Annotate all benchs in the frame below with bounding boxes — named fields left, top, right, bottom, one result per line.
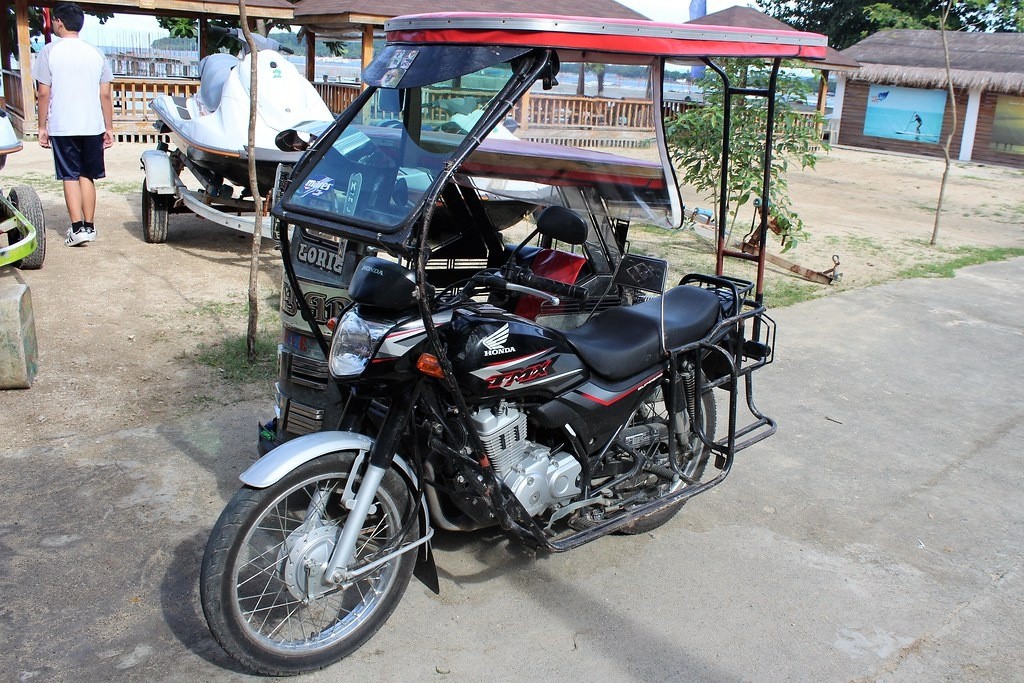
left=482, top=245, right=593, bottom=322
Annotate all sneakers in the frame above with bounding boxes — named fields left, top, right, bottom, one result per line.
left=64, top=226, right=89, bottom=247
left=85, top=226, right=97, bottom=241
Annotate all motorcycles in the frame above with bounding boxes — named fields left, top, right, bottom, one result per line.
left=0, top=61, right=25, bottom=172
left=147, top=22, right=337, bottom=197
left=195, top=8, right=831, bottom=678
left=370, top=97, right=560, bottom=233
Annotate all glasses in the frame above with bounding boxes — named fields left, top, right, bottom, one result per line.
left=52, top=18, right=57, bottom=23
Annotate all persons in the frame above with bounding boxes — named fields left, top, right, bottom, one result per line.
left=31, top=3, right=114, bottom=247
left=685, top=96, right=703, bottom=113
left=909, top=112, right=922, bottom=134
